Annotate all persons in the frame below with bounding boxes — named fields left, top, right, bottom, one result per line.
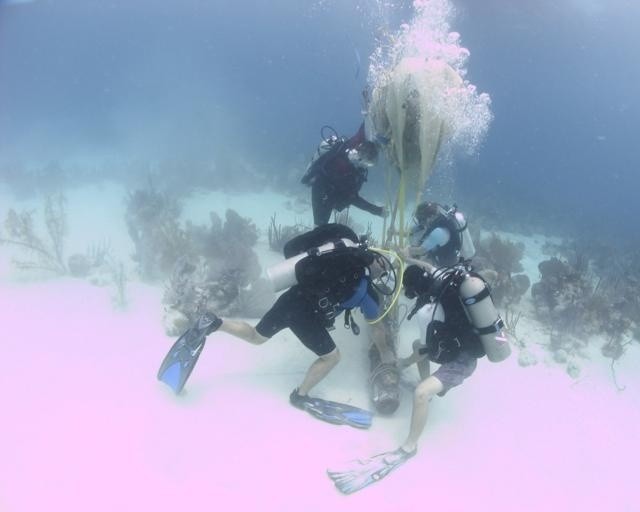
left=382, top=246, right=485, bottom=466
left=307, top=119, right=390, bottom=234
left=384, top=200, right=464, bottom=269
left=185, top=243, right=400, bottom=416
